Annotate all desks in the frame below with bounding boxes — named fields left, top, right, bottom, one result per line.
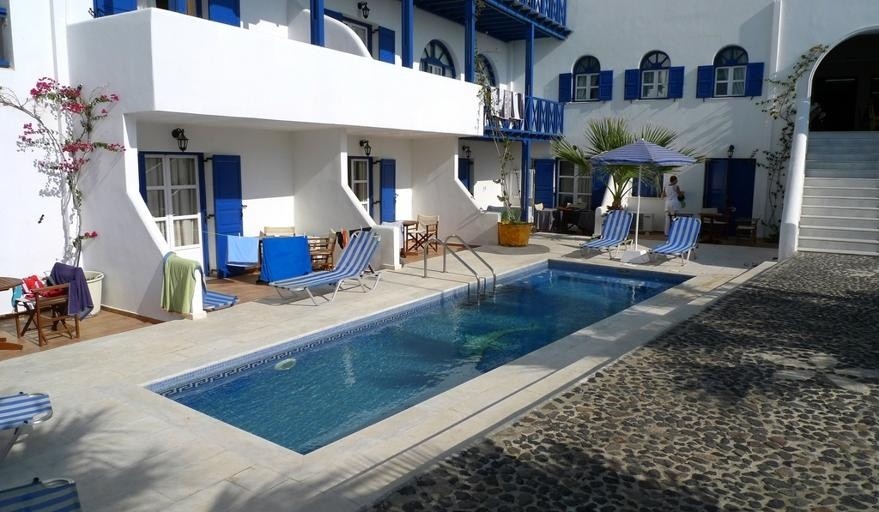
left=0, top=277, right=23, bottom=350
left=392, top=220, right=419, bottom=257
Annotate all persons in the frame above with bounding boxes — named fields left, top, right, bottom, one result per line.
left=659, top=175, right=685, bottom=237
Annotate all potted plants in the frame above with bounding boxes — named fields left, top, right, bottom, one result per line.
left=475, top=44, right=533, bottom=246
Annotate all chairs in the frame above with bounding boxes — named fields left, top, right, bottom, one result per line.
left=579, top=207, right=760, bottom=265
left=0, top=392, right=53, bottom=464
left=408, top=213, right=439, bottom=253
left=259, top=227, right=381, bottom=306
left=204, top=290, right=239, bottom=311
left=0, top=477, right=82, bottom=512
left=13, top=262, right=80, bottom=347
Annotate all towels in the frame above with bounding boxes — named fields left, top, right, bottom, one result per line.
left=48, top=263, right=93, bottom=321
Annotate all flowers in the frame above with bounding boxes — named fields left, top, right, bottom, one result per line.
left=17, top=76, right=126, bottom=267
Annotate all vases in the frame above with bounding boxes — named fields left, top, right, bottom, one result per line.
left=84, top=270, right=104, bottom=319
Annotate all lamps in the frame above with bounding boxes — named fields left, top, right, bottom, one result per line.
left=462, top=145, right=471, bottom=158
left=172, top=128, right=189, bottom=152
left=360, top=140, right=371, bottom=155
left=358, top=2, right=370, bottom=18
left=727, top=145, right=735, bottom=159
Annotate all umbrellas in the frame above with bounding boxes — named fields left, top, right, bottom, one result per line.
left=587, top=136, right=699, bottom=251
